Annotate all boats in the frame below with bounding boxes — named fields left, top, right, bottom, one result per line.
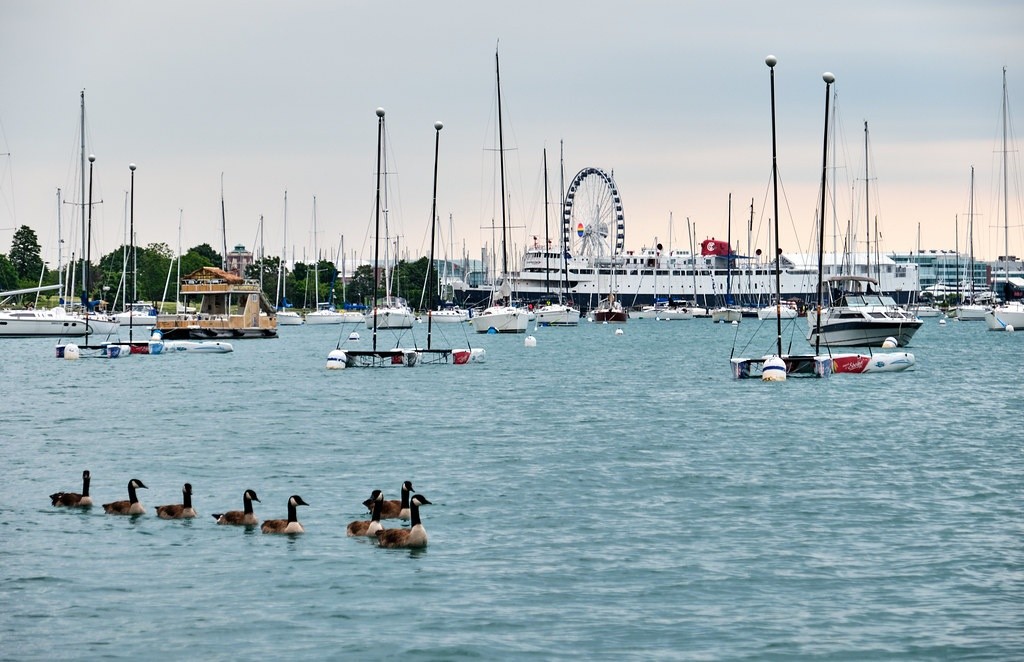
left=0, top=41, right=1023, bottom=382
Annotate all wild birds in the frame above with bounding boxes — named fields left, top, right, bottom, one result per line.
left=211, top=489, right=261, bottom=525
left=374, top=494, right=432, bottom=548
left=154, top=483, right=198, bottom=519
left=362, top=481, right=415, bottom=521
left=346, top=490, right=385, bottom=537
left=49, top=470, right=93, bottom=507
left=261, top=495, right=309, bottom=535
left=101, top=479, right=149, bottom=515
